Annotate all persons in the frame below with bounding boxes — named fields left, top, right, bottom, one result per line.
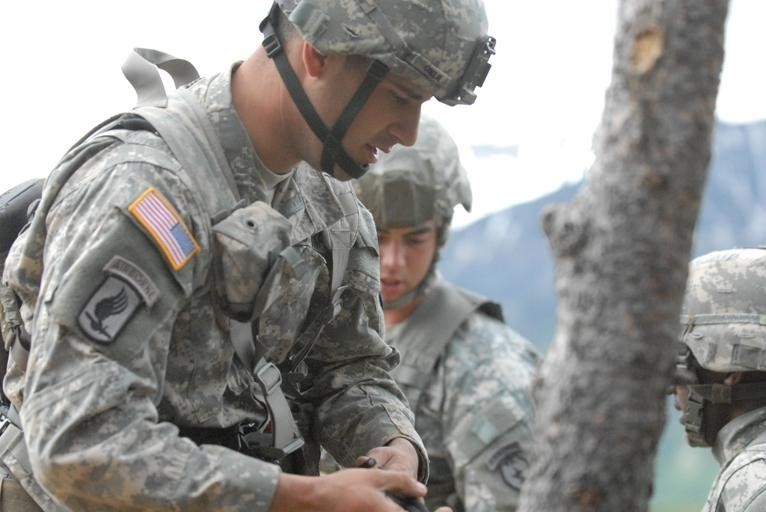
left=666, top=243, right=766, bottom=512
left=318, top=114, right=547, bottom=512
left=0, top=0, right=498, bottom=512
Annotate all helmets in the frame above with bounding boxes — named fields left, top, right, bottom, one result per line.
left=672, top=248, right=766, bottom=373
left=348, top=117, right=471, bottom=226
left=266, top=0, right=497, bottom=106
